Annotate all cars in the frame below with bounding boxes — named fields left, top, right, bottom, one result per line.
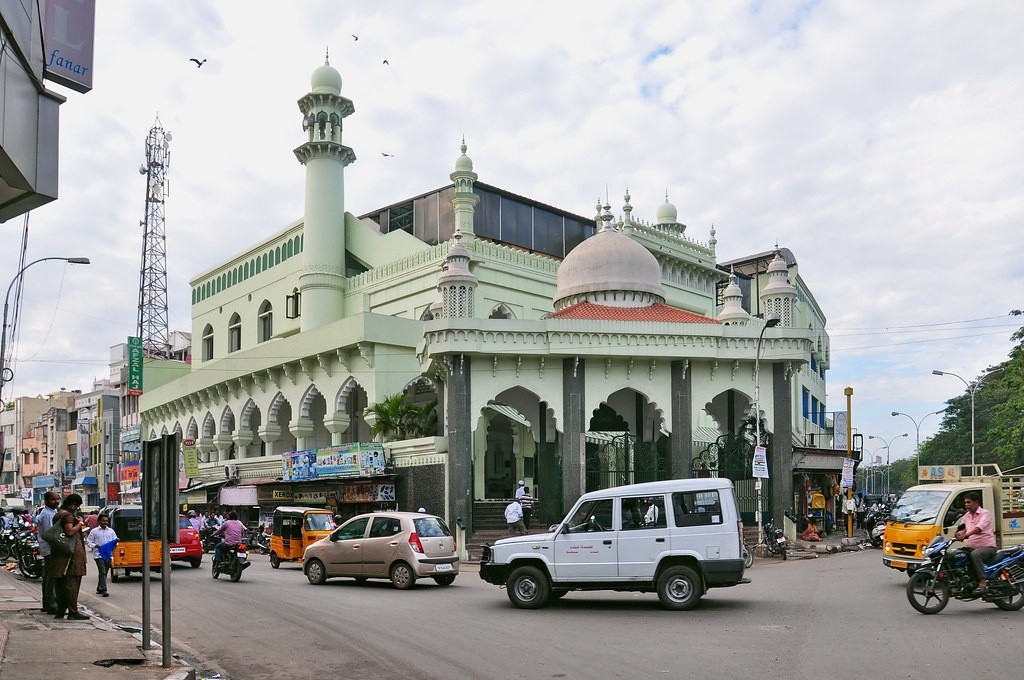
left=302, top=511, right=460, bottom=590
left=170, top=514, right=202, bottom=567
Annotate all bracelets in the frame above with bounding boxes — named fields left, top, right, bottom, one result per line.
left=79, top=522, right=84, bottom=526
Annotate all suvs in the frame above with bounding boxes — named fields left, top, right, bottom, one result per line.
left=478, top=477, right=752, bottom=612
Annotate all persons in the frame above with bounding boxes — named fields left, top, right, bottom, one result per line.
left=519, top=487, right=534, bottom=533
left=188, top=510, right=229, bottom=553
left=53, top=494, right=90, bottom=620
left=76, top=509, right=99, bottom=532
left=86, top=514, right=120, bottom=597
left=505, top=498, right=526, bottom=537
left=213, top=510, right=244, bottom=569
left=842, top=489, right=893, bottom=529
left=515, top=480, right=533, bottom=516
left=955, top=492, right=997, bottom=594
left=644, top=497, right=658, bottom=526
left=694, top=505, right=705, bottom=512
left=35, top=491, right=69, bottom=614
left=0, top=507, right=43, bottom=530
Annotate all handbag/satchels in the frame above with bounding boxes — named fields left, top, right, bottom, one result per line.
left=41, top=513, right=76, bottom=554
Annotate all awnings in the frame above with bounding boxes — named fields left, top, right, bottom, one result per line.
left=486, top=404, right=769, bottom=447
left=116, top=479, right=227, bottom=494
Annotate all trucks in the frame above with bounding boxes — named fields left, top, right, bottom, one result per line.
left=882, top=464, right=1024, bottom=585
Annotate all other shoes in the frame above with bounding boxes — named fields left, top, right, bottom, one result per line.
left=972, top=585, right=986, bottom=594
left=96, top=590, right=102, bottom=594
left=103, top=593, right=109, bottom=596
left=67, top=610, right=90, bottom=620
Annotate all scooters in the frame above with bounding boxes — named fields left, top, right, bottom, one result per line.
left=0, top=505, right=47, bottom=579
left=861, top=493, right=899, bottom=549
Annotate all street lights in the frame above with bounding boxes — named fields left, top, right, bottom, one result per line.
left=868, top=433, right=909, bottom=496
left=0, top=257, right=90, bottom=390
left=863, top=446, right=892, bottom=496
left=755, top=314, right=782, bottom=545
left=932, top=367, right=1004, bottom=474
left=891, top=411, right=942, bottom=484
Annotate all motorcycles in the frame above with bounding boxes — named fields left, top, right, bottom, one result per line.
left=269, top=506, right=336, bottom=569
left=200, top=521, right=273, bottom=582
left=907, top=523, right=1024, bottom=615
left=761, top=515, right=788, bottom=561
left=106, top=505, right=171, bottom=584
